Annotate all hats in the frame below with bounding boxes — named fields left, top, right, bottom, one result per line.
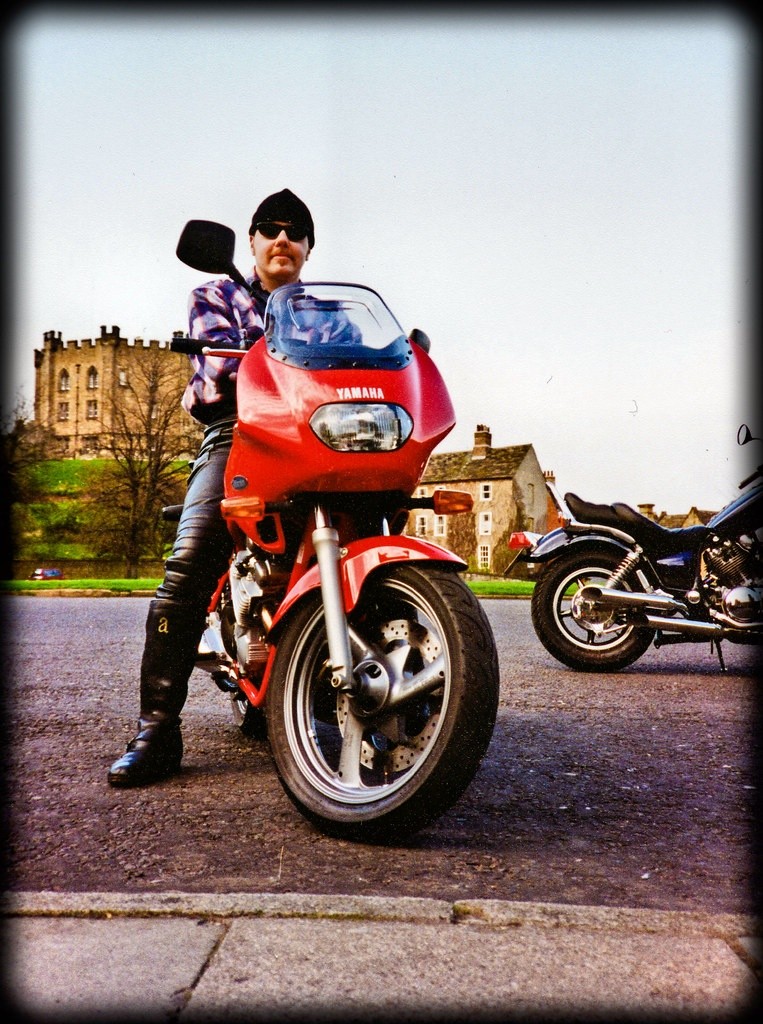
left=252, top=188, right=315, bottom=249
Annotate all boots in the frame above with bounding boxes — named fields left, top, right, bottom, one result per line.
left=107, top=600, right=205, bottom=784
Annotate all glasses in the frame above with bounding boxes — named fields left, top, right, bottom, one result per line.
left=253, top=221, right=311, bottom=249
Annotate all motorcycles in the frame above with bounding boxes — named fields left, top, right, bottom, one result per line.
left=501, top=423, right=762, bottom=674
left=158, top=218, right=503, bottom=847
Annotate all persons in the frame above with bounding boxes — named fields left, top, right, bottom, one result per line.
left=107, top=186, right=363, bottom=791
